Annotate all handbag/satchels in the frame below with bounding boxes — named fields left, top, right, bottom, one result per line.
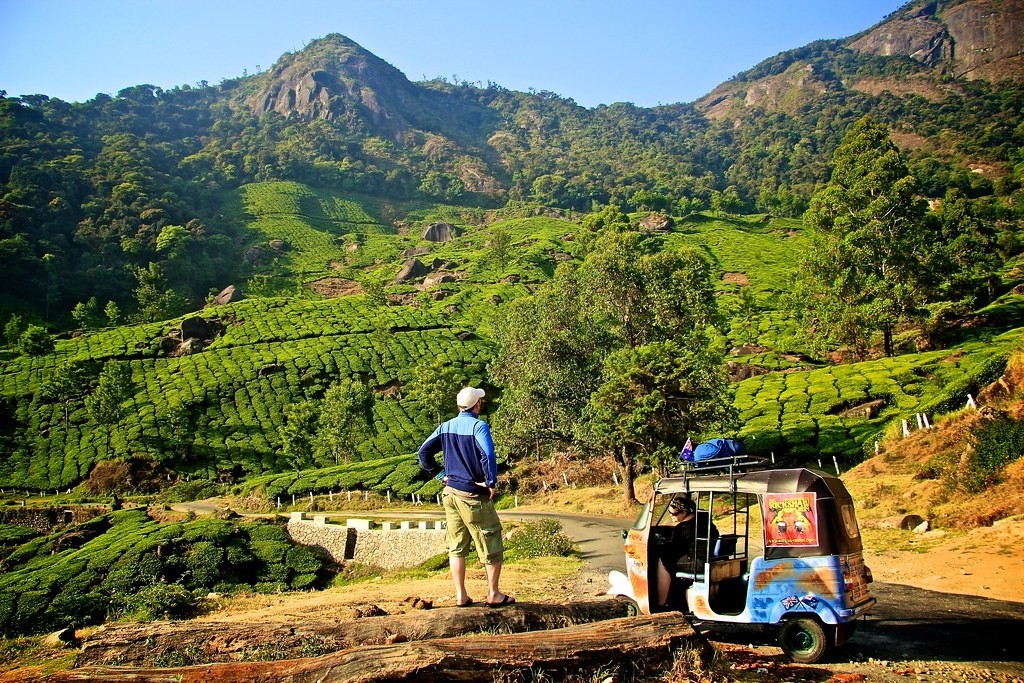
left=677, top=555, right=705, bottom=574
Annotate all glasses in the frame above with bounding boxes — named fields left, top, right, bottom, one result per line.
left=668, top=510, right=683, bottom=516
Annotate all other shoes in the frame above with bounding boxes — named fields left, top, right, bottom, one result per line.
left=650, top=603, right=671, bottom=613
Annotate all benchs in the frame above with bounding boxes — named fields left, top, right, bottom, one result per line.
left=675, top=533, right=737, bottom=580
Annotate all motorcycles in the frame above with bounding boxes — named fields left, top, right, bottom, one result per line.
left=606, top=455, right=879, bottom=666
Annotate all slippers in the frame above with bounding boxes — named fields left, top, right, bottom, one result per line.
left=457, top=596, right=472, bottom=607
left=486, top=595, right=515, bottom=608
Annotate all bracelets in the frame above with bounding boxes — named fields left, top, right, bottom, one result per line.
left=435, top=470, right=445, bottom=483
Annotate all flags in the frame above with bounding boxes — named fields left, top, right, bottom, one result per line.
left=680, top=438, right=694, bottom=463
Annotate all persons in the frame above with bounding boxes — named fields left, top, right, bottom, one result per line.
left=417, top=386, right=515, bottom=607
left=655, top=497, right=720, bottom=611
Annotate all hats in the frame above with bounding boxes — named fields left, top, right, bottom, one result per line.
left=457, top=387, right=485, bottom=412
